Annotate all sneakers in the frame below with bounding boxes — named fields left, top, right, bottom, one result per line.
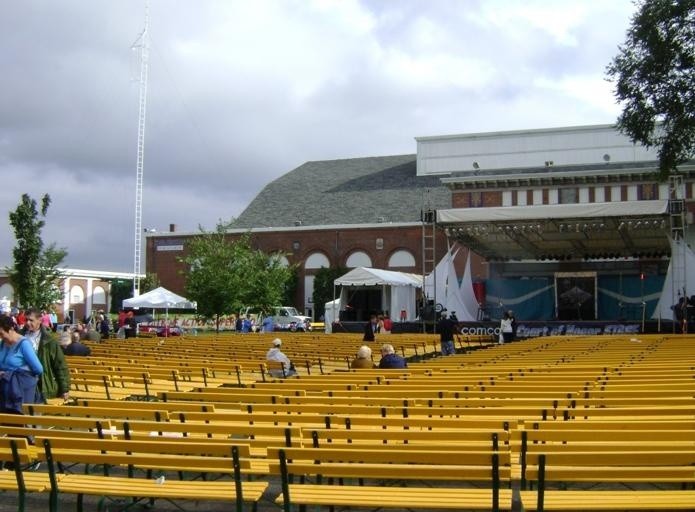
left=33, top=462, right=40, bottom=470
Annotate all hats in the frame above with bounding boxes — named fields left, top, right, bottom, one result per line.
left=272, top=338, right=281, bottom=346
left=358, top=346, right=371, bottom=357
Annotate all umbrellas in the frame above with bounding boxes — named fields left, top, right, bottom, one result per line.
left=122, top=286, right=198, bottom=338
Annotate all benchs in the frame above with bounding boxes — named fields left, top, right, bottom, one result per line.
left=0, top=333, right=695, bottom=507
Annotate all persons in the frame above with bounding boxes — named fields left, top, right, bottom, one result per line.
left=376, top=315, right=385, bottom=334
left=674, top=297, right=687, bottom=334
left=262, top=314, right=274, bottom=333
left=66, top=331, right=92, bottom=357
left=363, top=315, right=377, bottom=342
left=305, top=320, right=312, bottom=332
left=498, top=313, right=513, bottom=344
left=56, top=326, right=72, bottom=348
left=290, top=321, right=296, bottom=333
left=450, top=310, right=458, bottom=330
left=508, top=310, right=517, bottom=343
left=297, top=319, right=307, bottom=332
left=0, top=314, right=44, bottom=472
left=350, top=344, right=378, bottom=369
left=321, top=314, right=326, bottom=322
left=436, top=312, right=458, bottom=355
left=80, top=323, right=91, bottom=340
left=236, top=317, right=244, bottom=333
left=98, top=312, right=110, bottom=338
left=124, top=311, right=137, bottom=339
left=241, top=315, right=251, bottom=333
left=76, top=322, right=84, bottom=335
left=266, top=339, right=298, bottom=378
left=15, top=309, right=70, bottom=464
left=382, top=312, right=393, bottom=333
left=40, top=310, right=54, bottom=329
left=686, top=295, right=695, bottom=334
left=380, top=343, right=407, bottom=369
left=117, top=309, right=127, bottom=336
left=424, top=299, right=436, bottom=334
left=49, top=310, right=59, bottom=332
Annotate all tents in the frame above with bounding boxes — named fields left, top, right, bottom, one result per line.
left=333, top=266, right=433, bottom=322
left=325, top=298, right=340, bottom=333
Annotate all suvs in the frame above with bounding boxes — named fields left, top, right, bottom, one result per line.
left=244, top=306, right=314, bottom=333
